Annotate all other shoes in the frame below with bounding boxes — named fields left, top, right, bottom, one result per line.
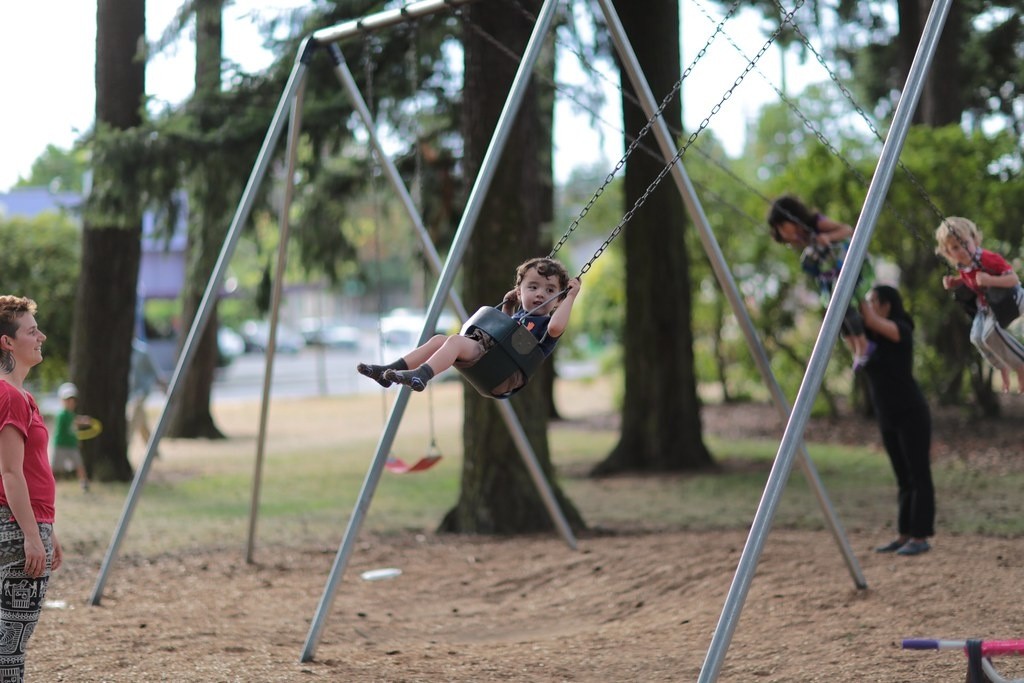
left=877, top=539, right=909, bottom=551
left=897, top=541, right=929, bottom=554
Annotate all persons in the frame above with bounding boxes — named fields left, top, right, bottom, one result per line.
left=48, top=382, right=98, bottom=501
left=934, top=216, right=1024, bottom=394
left=125, top=339, right=169, bottom=458
left=357, top=257, right=582, bottom=400
left=0, top=292, right=64, bottom=683
left=767, top=197, right=937, bottom=556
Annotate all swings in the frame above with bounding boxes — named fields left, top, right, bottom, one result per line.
left=696, top=0, right=1019, bottom=333
left=443, top=1, right=874, bottom=301
left=457, top=0, right=811, bottom=398
left=361, top=22, right=443, bottom=475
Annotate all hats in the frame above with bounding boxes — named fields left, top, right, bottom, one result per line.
left=58, top=381, right=78, bottom=400
